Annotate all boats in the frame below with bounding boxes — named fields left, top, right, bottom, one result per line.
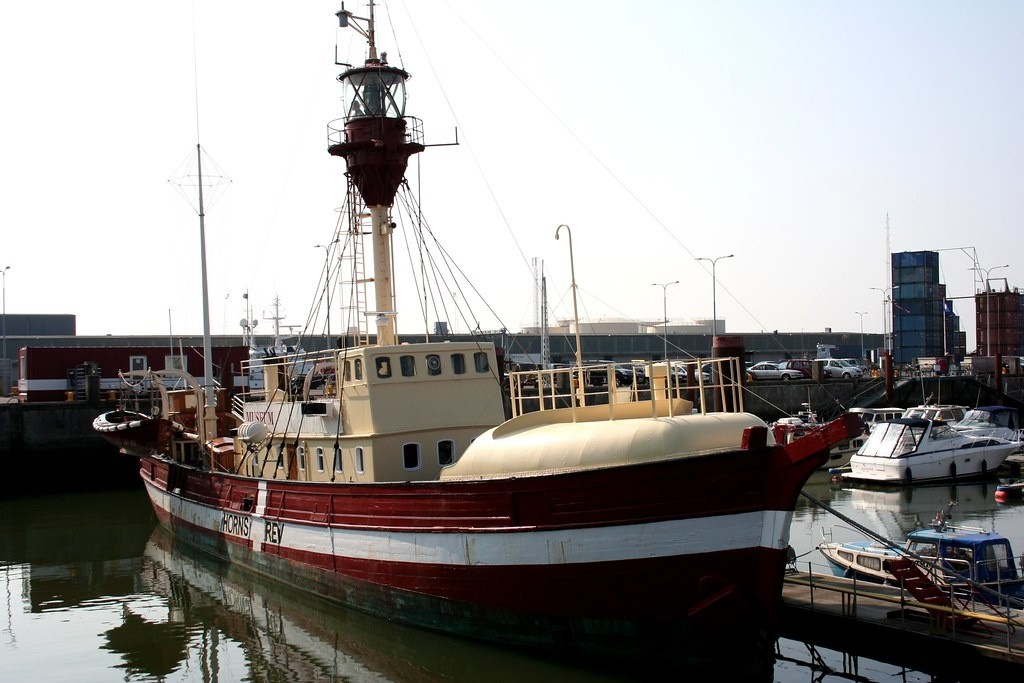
left=787, top=393, right=1024, bottom=454
left=92, top=410, right=184, bottom=458
left=829, top=418, right=1024, bottom=486
left=818, top=502, right=1024, bottom=611
left=139, top=523, right=608, bottom=683
left=118, top=0, right=865, bottom=670
left=842, top=478, right=1010, bottom=543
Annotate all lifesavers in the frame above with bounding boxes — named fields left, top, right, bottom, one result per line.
left=129, top=381, right=144, bottom=396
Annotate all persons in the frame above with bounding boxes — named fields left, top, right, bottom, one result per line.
left=515, top=366, right=524, bottom=396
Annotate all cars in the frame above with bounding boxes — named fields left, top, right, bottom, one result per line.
left=814, top=359, right=863, bottom=379
left=700, top=363, right=757, bottom=386
left=572, top=360, right=639, bottom=386
left=670, top=366, right=710, bottom=383
left=785, top=359, right=813, bottom=378
left=746, top=363, right=803, bottom=380
left=842, top=359, right=868, bottom=372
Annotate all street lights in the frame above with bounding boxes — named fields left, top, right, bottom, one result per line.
left=855, top=312, right=868, bottom=357
left=870, top=286, right=899, bottom=351
left=554, top=224, right=585, bottom=406
left=652, top=281, right=679, bottom=360
left=314, top=239, right=340, bottom=350
left=696, top=255, right=734, bottom=336
left=0, top=266, right=10, bottom=397
left=968, top=265, right=1009, bottom=357
